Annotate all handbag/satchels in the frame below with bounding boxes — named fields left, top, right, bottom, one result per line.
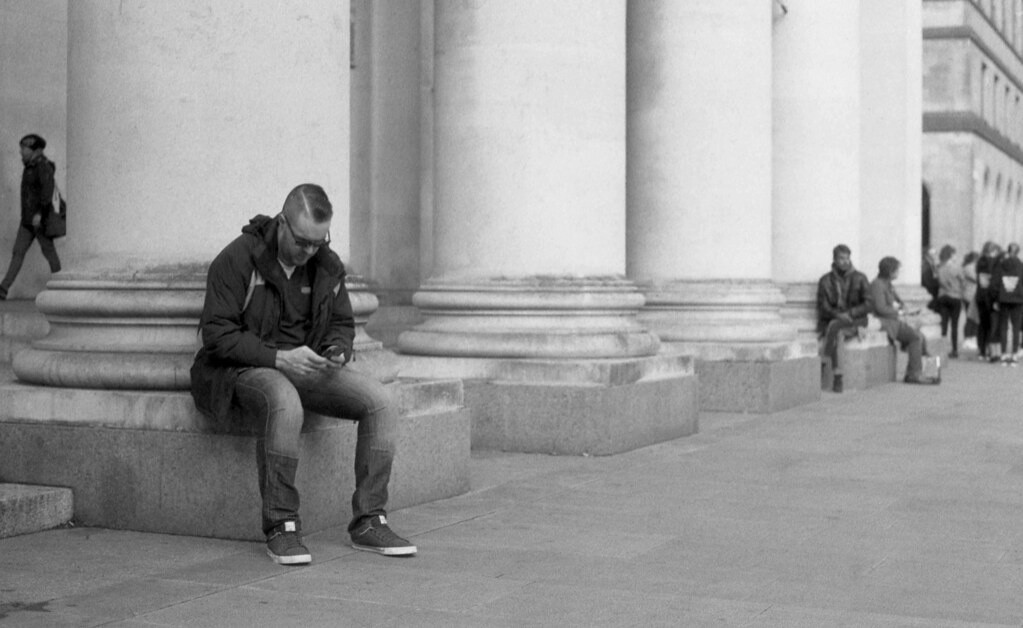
left=42, top=193, right=67, bottom=237
left=920, top=354, right=941, bottom=383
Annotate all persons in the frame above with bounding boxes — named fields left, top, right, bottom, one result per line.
left=922, top=241, right=1023, bottom=366
left=816, top=244, right=873, bottom=392
left=870, top=256, right=941, bottom=384
left=190, top=184, right=417, bottom=565
left=0, top=134, right=61, bottom=300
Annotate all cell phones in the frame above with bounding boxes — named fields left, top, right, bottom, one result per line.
left=326, top=345, right=348, bottom=360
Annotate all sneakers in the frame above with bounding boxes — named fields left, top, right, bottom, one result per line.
left=264, top=520, right=311, bottom=564
left=349, top=514, right=416, bottom=555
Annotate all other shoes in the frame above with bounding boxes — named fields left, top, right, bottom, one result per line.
left=905, top=374, right=933, bottom=384
left=948, top=350, right=959, bottom=358
left=0, top=286, right=8, bottom=299
left=941, top=324, right=947, bottom=336
left=832, top=374, right=842, bottom=392
left=976, top=349, right=1023, bottom=363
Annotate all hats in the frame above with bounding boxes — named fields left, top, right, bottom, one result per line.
left=20, top=133, right=47, bottom=151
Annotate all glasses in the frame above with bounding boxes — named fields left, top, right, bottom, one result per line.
left=284, top=215, right=331, bottom=251
